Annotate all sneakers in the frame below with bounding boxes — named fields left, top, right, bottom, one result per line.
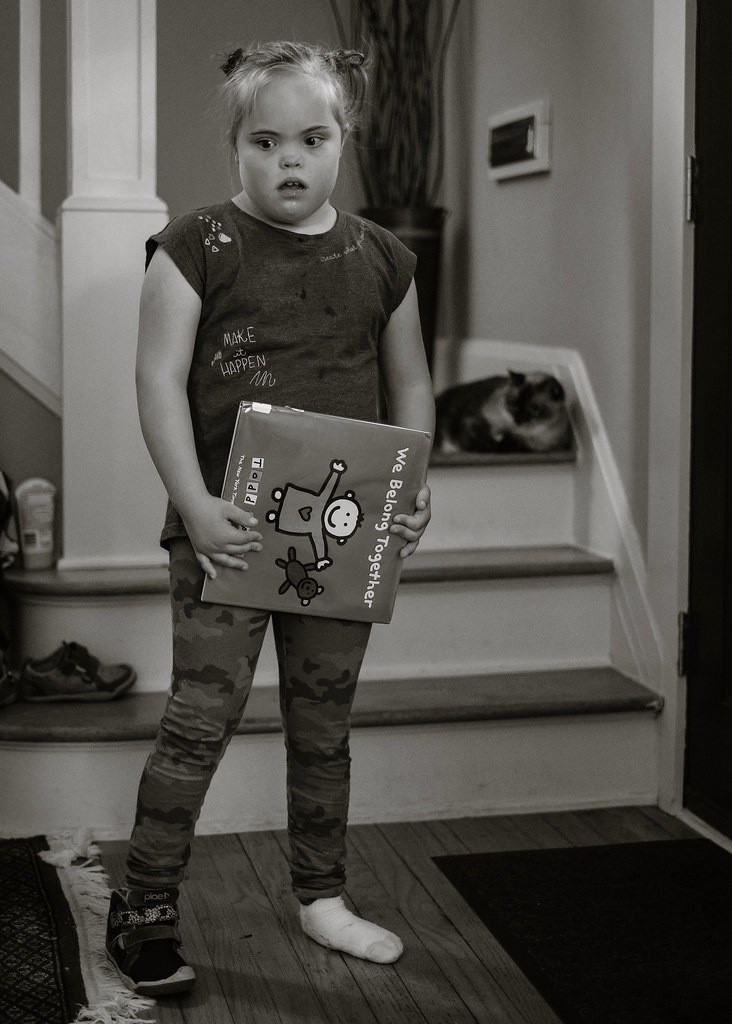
left=20, top=640, right=136, bottom=703
left=0, top=666, right=16, bottom=706
left=106, top=887, right=196, bottom=996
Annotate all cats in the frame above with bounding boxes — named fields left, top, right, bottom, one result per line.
left=431, top=367, right=570, bottom=452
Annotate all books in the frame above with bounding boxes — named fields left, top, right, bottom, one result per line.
left=201, top=400, right=433, bottom=625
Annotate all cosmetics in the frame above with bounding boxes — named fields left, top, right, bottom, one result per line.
left=15, top=477, right=54, bottom=572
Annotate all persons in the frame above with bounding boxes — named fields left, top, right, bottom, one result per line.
left=106, top=38, right=436, bottom=992
